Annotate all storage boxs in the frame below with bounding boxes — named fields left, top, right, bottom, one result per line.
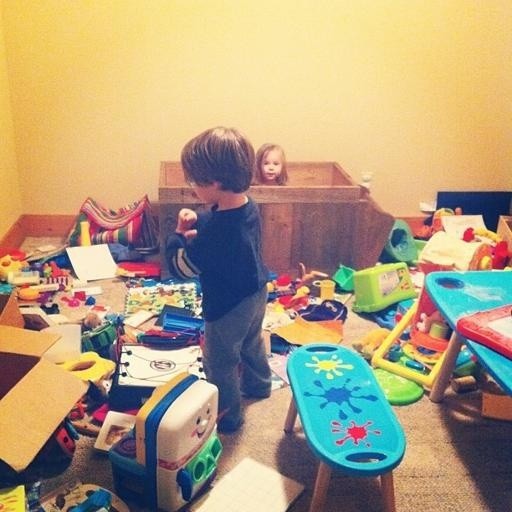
left=156, top=152, right=399, bottom=281
left=0, top=322, right=89, bottom=475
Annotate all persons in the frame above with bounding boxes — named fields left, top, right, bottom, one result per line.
left=165, top=126, right=272, bottom=434
left=252, top=143, right=289, bottom=187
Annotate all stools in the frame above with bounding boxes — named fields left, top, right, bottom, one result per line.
left=281, top=341, right=406, bottom=511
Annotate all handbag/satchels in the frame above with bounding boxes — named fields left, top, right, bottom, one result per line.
left=68, top=197, right=160, bottom=256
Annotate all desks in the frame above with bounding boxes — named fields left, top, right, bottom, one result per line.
left=423, top=269, right=512, bottom=404
